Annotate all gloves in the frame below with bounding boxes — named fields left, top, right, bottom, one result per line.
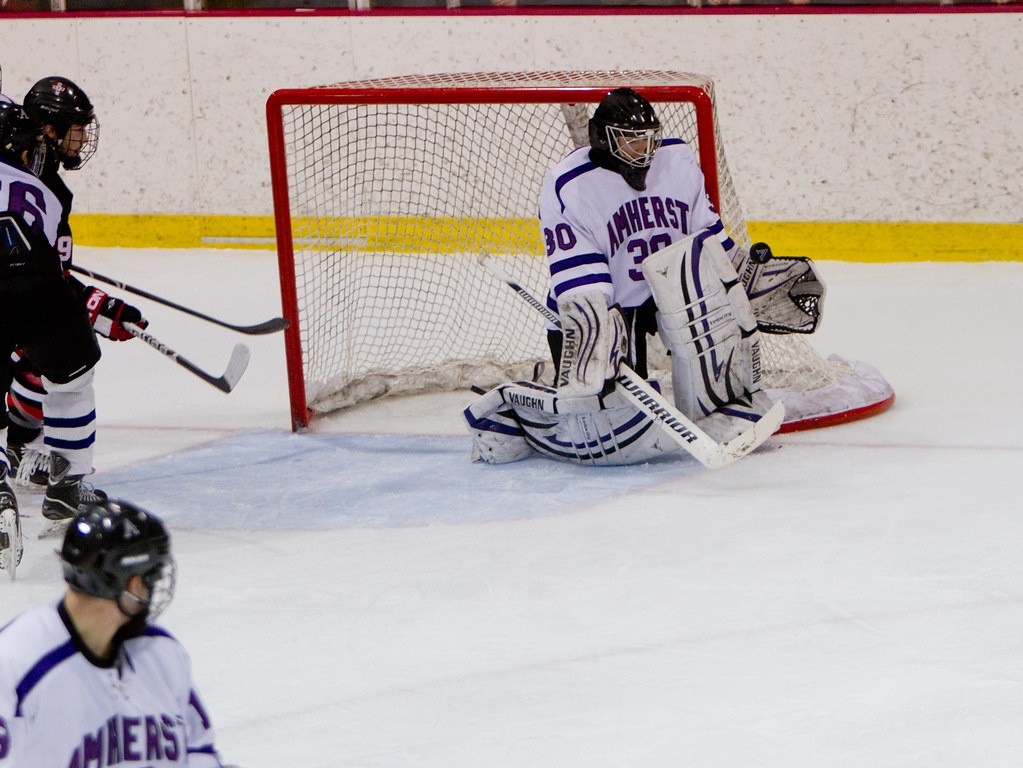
left=83, top=286, right=149, bottom=342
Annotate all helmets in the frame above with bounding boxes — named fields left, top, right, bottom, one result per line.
left=60, top=499, right=176, bottom=623
left=588, top=88, right=663, bottom=192
left=0, top=101, right=47, bottom=177
left=24, top=77, right=100, bottom=169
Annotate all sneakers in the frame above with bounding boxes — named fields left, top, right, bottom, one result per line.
left=0, top=461, right=24, bottom=579
left=38, top=449, right=108, bottom=541
left=5, top=411, right=51, bottom=492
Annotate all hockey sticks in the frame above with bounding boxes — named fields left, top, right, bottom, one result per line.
left=476, top=250, right=787, bottom=471
left=68, top=263, right=292, bottom=337
left=121, top=319, right=251, bottom=394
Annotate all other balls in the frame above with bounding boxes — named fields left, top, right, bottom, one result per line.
left=749, top=242, right=772, bottom=264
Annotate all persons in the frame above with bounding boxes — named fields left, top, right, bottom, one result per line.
left=0, top=498, right=231, bottom=768
left=463, top=86, right=823, bottom=466
left=0, top=77, right=147, bottom=582
